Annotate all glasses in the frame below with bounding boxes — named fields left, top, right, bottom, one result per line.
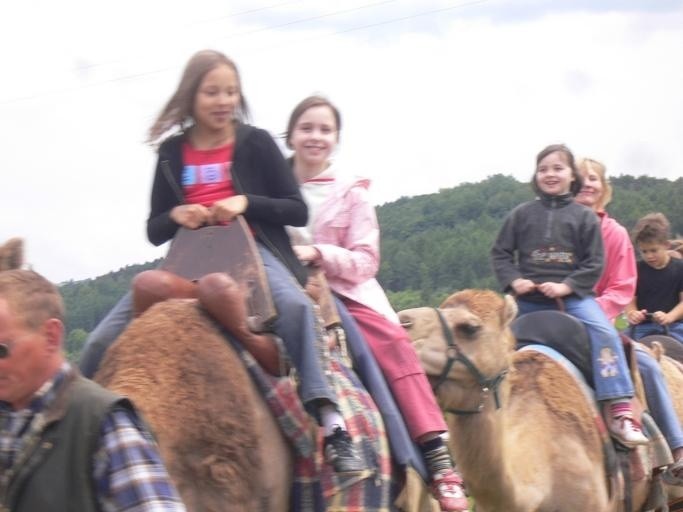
left=0, top=333, right=36, bottom=360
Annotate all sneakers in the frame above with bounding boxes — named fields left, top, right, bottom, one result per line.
left=609, top=414, right=651, bottom=446
left=322, top=425, right=364, bottom=473
left=431, top=468, right=468, bottom=512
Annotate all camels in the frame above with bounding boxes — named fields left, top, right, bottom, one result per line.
left=393, top=287, right=683, bottom=510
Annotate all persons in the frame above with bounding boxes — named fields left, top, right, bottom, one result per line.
left=620, top=212, right=683, bottom=345
left=75, top=48, right=367, bottom=476
left=275, top=94, right=469, bottom=511
left=572, top=156, right=683, bottom=475
left=0, top=268, right=188, bottom=511
left=490, top=142, right=651, bottom=448
